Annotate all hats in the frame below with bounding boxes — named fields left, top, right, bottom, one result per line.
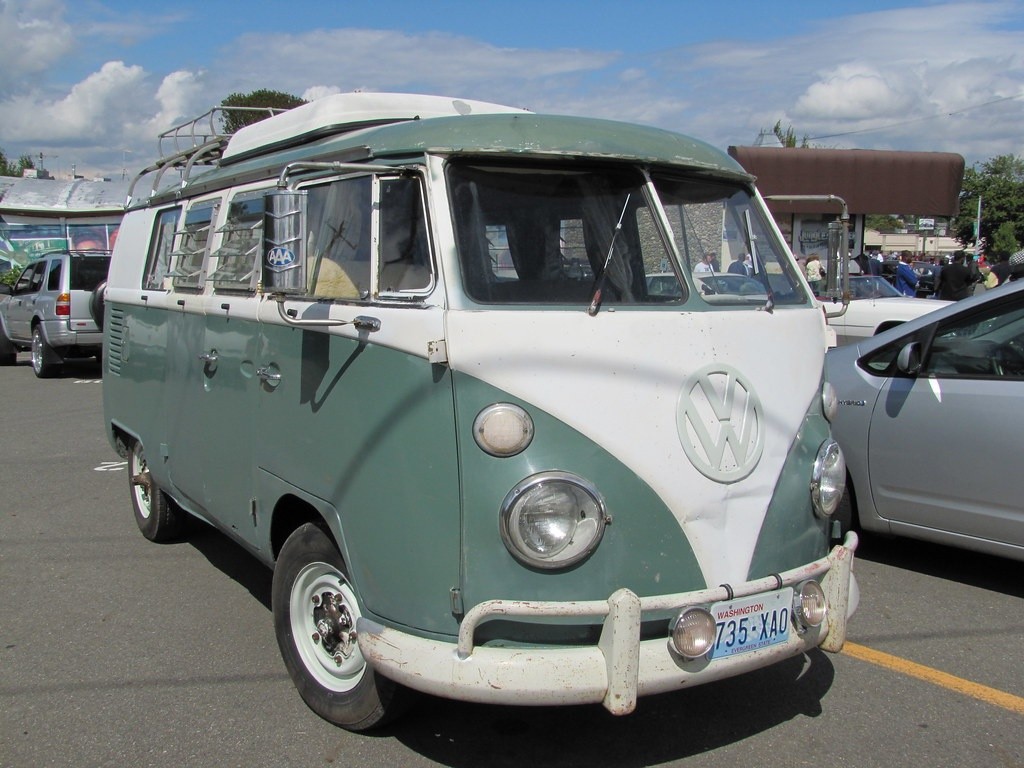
left=1009, top=251, right=1024, bottom=266
left=953, top=250, right=966, bottom=258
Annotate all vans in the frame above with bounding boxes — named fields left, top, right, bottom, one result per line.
left=95, top=90, right=866, bottom=734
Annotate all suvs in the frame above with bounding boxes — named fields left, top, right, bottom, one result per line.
left=0, top=246, right=114, bottom=379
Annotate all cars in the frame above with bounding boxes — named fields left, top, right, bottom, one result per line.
left=790, top=273, right=959, bottom=339
left=825, top=274, right=1023, bottom=562
left=644, top=271, right=770, bottom=304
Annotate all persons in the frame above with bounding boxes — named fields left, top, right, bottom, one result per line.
left=868, top=246, right=1024, bottom=301
left=791, top=253, right=825, bottom=297
left=72, top=230, right=107, bottom=254
left=694, top=252, right=752, bottom=277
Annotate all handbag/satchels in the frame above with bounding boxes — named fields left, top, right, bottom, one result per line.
left=819, top=261, right=826, bottom=277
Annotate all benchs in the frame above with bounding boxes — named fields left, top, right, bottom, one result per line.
left=306, top=257, right=430, bottom=300
